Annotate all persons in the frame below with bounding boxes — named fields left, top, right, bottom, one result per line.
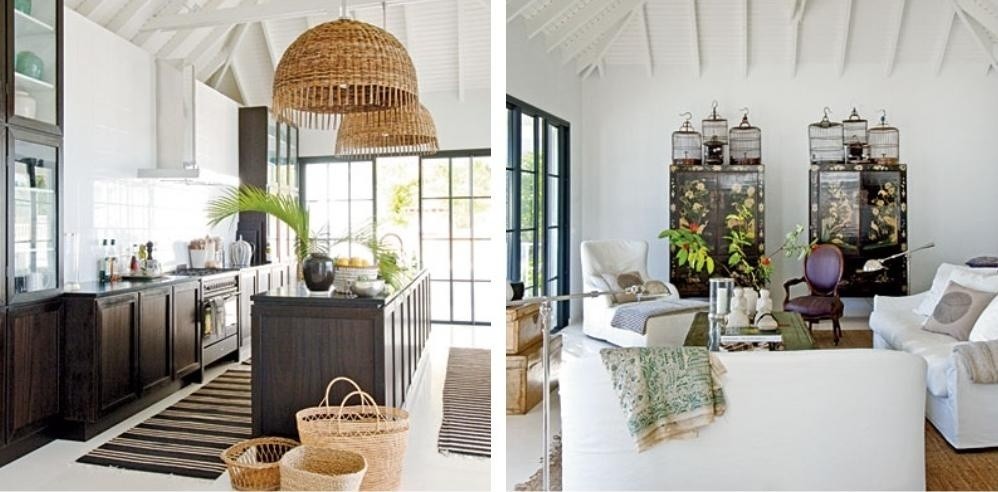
left=728, top=287, right=749, bottom=324
left=756, top=289, right=772, bottom=323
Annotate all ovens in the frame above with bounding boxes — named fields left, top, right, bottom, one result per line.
left=202, top=288, right=238, bottom=348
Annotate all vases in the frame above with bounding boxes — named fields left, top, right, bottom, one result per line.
left=737, top=286, right=758, bottom=321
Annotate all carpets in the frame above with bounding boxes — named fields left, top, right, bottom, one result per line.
left=434, top=344, right=491, bottom=461
left=74, top=369, right=253, bottom=480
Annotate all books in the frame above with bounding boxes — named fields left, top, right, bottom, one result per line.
left=721, top=325, right=783, bottom=342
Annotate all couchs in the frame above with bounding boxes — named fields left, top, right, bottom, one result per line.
left=559, top=350, right=928, bottom=490
left=867, top=254, right=998, bottom=453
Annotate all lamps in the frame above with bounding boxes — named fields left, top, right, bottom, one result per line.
left=333, top=1, right=439, bottom=160
left=273, top=1, right=418, bottom=130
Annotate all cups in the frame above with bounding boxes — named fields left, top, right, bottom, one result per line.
left=14, top=273, right=29, bottom=294
left=27, top=272, right=50, bottom=292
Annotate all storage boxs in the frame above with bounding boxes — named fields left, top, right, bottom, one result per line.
left=506, top=299, right=563, bottom=415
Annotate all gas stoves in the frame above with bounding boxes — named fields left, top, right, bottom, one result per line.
left=164, top=267, right=239, bottom=295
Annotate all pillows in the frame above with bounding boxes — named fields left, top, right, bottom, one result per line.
left=592, top=267, right=680, bottom=304
left=922, top=279, right=998, bottom=341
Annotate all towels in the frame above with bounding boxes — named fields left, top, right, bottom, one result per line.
left=599, top=346, right=727, bottom=452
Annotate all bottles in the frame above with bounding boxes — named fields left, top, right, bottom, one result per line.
left=98, top=238, right=162, bottom=287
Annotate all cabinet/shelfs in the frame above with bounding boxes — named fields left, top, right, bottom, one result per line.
left=668, top=164, right=908, bottom=299
left=167, top=281, right=201, bottom=392
left=58, top=294, right=138, bottom=442
left=0, top=0, right=64, bottom=468
left=238, top=106, right=299, bottom=363
left=133, top=286, right=173, bottom=414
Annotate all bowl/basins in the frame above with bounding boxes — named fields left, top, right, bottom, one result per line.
left=333, top=266, right=379, bottom=292
left=346, top=279, right=385, bottom=298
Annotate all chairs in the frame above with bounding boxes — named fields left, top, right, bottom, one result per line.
left=581, top=237, right=719, bottom=349
left=783, top=241, right=845, bottom=346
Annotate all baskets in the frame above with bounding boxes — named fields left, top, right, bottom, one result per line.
left=219, top=437, right=300, bottom=491
left=279, top=446, right=367, bottom=490
left=294, top=377, right=410, bottom=490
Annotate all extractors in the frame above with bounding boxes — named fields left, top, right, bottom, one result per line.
left=138, top=62, right=241, bottom=187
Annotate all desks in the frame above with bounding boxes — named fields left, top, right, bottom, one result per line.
left=682, top=312, right=817, bottom=350
left=250, top=267, right=430, bottom=439
